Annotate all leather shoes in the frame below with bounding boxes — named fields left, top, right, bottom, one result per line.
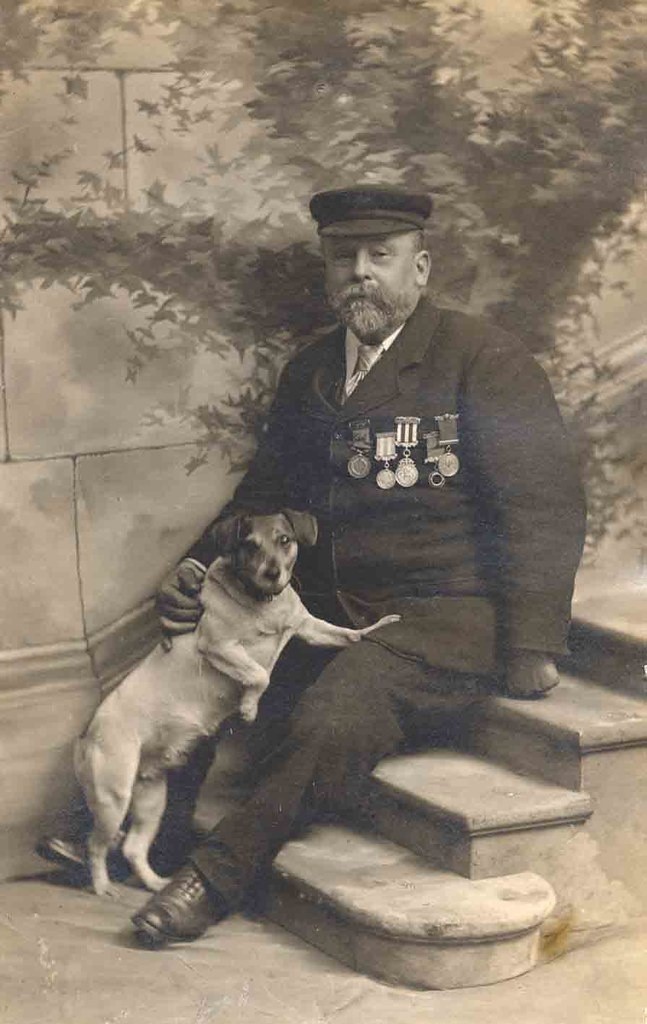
left=131, top=857, right=225, bottom=944
left=36, top=832, right=184, bottom=872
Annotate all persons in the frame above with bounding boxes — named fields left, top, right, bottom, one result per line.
left=37, top=185, right=588, bottom=943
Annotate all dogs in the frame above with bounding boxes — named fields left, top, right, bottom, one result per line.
left=71, top=506, right=403, bottom=901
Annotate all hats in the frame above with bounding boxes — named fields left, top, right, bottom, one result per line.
left=309, top=187, right=432, bottom=236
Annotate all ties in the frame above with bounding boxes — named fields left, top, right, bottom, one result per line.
left=342, top=345, right=383, bottom=407
